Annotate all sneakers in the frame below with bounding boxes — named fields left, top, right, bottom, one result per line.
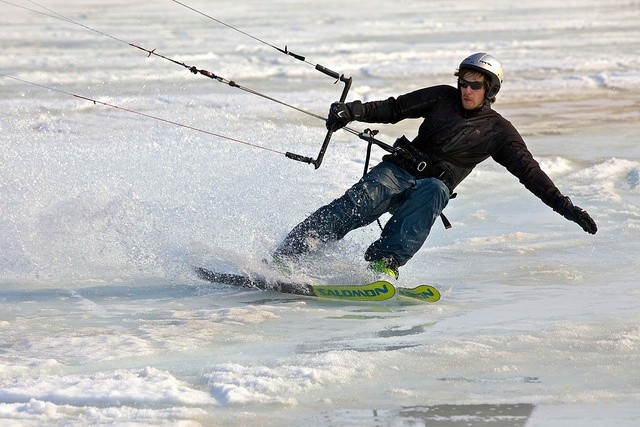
left=367, top=258, right=400, bottom=281
left=263, top=255, right=295, bottom=280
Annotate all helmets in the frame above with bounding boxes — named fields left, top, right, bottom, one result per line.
left=454, top=53, right=504, bottom=96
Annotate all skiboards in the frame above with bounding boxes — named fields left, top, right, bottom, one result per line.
left=187, top=265, right=442, bottom=304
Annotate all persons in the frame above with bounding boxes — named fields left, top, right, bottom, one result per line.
left=260, top=50, right=597, bottom=281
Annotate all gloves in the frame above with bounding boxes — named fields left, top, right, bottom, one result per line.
left=326, top=100, right=364, bottom=133
left=553, top=195, right=598, bottom=235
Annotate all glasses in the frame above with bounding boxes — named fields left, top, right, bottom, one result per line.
left=458, top=78, right=484, bottom=89
